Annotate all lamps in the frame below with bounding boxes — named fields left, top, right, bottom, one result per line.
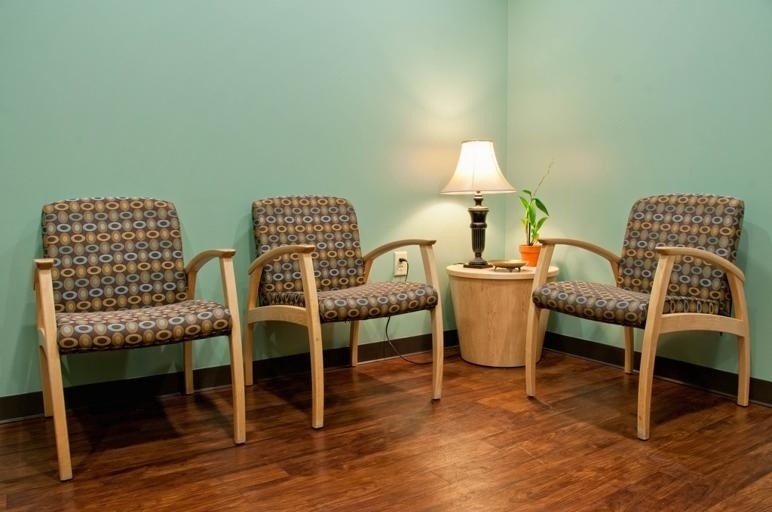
left=437, top=137, right=517, bottom=270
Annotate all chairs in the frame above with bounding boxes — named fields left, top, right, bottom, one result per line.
left=238, top=190, right=446, bottom=429
left=520, top=189, right=755, bottom=442
left=27, top=190, right=249, bottom=482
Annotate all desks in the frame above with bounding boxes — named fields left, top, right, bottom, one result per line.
left=446, top=260, right=564, bottom=371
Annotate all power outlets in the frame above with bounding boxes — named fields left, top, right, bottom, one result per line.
left=393, top=248, right=410, bottom=278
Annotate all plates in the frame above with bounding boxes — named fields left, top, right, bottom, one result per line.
left=486, top=259, right=529, bottom=267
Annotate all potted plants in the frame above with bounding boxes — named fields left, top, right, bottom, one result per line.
left=512, top=185, right=552, bottom=267
